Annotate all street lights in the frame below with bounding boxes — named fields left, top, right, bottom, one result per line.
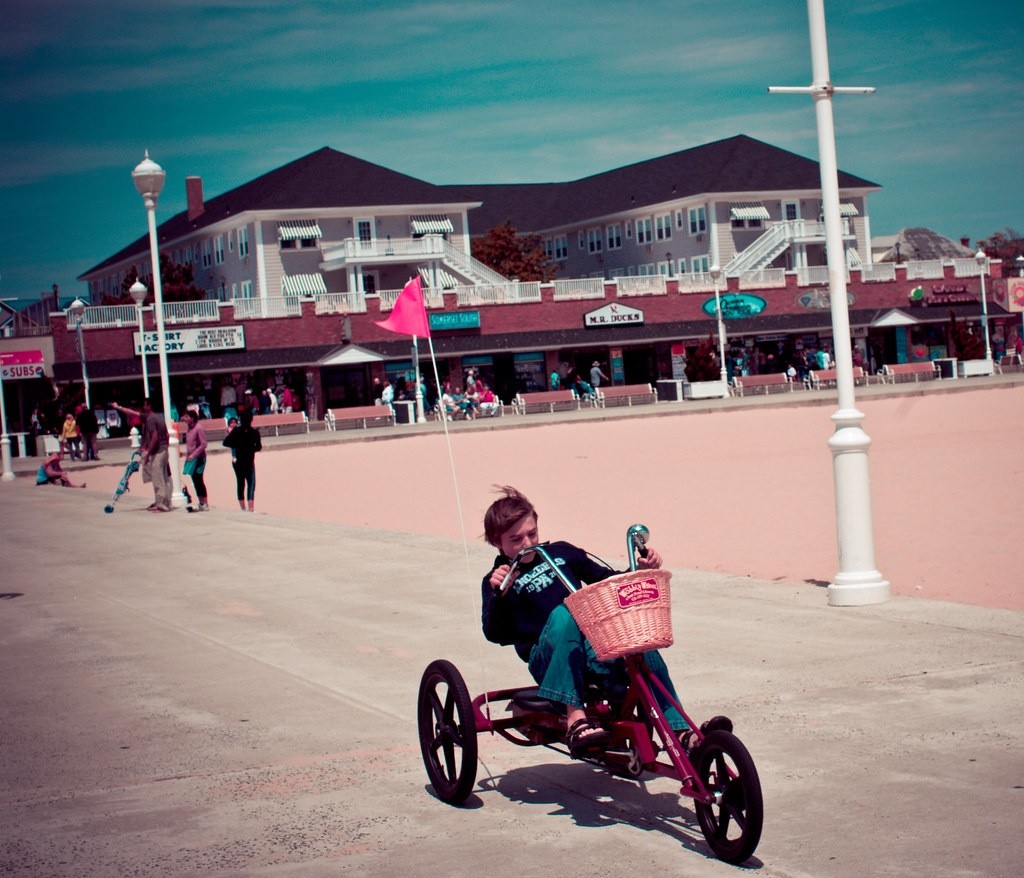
left=129, top=278, right=150, bottom=402
left=710, top=265, right=732, bottom=398
left=973, top=250, right=996, bottom=375
left=69, top=296, right=90, bottom=409
left=131, top=157, right=184, bottom=507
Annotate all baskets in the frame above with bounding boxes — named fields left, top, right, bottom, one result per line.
left=564, top=569, right=674, bottom=660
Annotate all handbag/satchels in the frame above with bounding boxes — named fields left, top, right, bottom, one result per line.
left=142, top=455, right=155, bottom=484
left=182, top=459, right=197, bottom=476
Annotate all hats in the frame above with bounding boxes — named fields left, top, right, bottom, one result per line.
left=592, top=361, right=600, bottom=367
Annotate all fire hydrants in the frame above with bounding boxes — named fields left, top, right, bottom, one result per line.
left=126, top=425, right=142, bottom=448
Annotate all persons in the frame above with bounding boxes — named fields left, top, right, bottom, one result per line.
left=374, top=369, right=499, bottom=422
left=707, top=341, right=864, bottom=388
left=225, top=417, right=239, bottom=461
left=177, top=374, right=295, bottom=421
left=29, top=397, right=173, bottom=510
left=481, top=483, right=732, bottom=755
left=223, top=409, right=263, bottom=512
left=550, top=358, right=609, bottom=403
left=184, top=410, right=209, bottom=510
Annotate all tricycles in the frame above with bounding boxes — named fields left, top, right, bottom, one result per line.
left=416, top=522, right=765, bottom=864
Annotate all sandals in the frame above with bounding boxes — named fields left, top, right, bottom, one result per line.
left=680, top=716, right=733, bottom=758
left=564, top=717, right=608, bottom=760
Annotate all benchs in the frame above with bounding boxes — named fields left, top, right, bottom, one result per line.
left=729, top=372, right=794, bottom=398
left=803, top=367, right=869, bottom=391
left=251, top=411, right=309, bottom=437
left=324, top=402, right=396, bottom=432
left=877, top=361, right=942, bottom=386
left=511, top=389, right=581, bottom=416
left=175, top=417, right=228, bottom=434
left=590, top=382, right=658, bottom=408
left=434, top=394, right=505, bottom=421
left=993, top=354, right=1024, bottom=375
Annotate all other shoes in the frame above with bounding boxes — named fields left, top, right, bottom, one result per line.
left=195, top=503, right=209, bottom=513
left=146, top=504, right=165, bottom=511
